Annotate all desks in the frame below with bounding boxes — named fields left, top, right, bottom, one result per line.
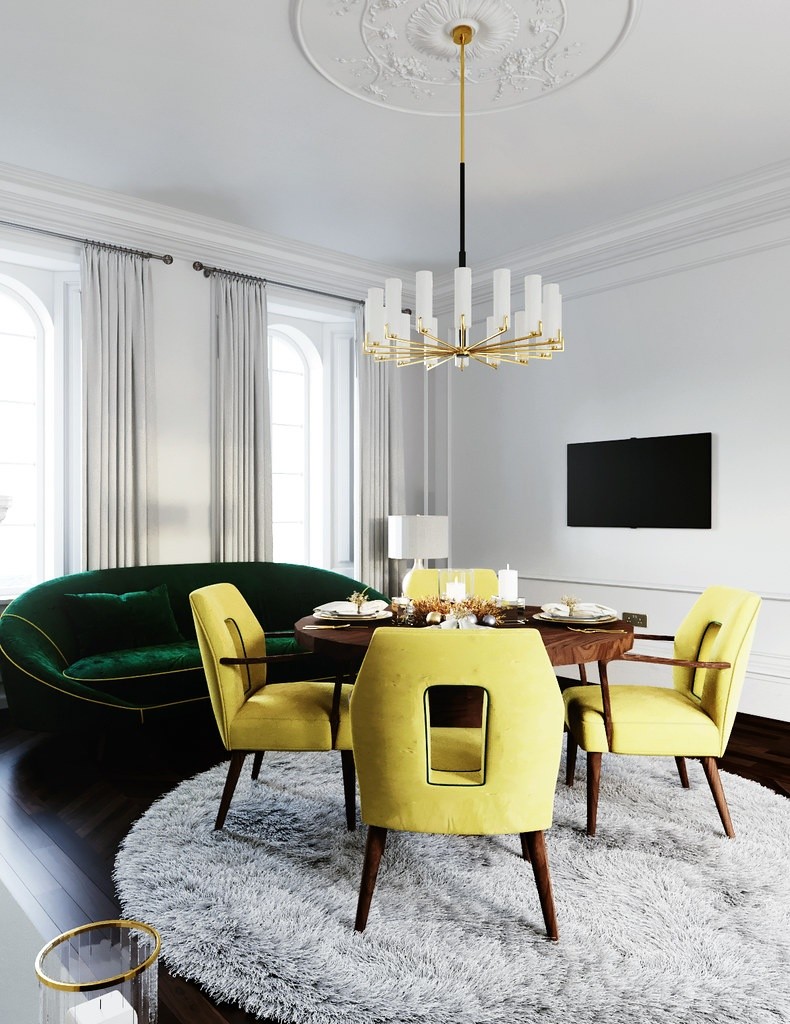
left=293, top=607, right=634, bottom=667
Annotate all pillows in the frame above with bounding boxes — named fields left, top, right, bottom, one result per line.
left=64, top=584, right=185, bottom=661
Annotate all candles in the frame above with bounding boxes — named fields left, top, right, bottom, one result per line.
left=446, top=575, right=466, bottom=601
left=499, top=564, right=519, bottom=600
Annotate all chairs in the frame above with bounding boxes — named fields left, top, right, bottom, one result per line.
left=188, top=582, right=357, bottom=832
left=348, top=627, right=565, bottom=941
left=402, top=568, right=499, bottom=600
left=562, top=586, right=762, bottom=838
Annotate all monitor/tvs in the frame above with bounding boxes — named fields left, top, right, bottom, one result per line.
left=567, top=432, right=713, bottom=530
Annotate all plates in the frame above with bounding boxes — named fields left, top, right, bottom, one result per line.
left=532, top=603, right=619, bottom=624
left=313, top=600, right=393, bottom=621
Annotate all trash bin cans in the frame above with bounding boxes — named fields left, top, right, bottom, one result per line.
left=34, top=920, right=161, bottom=1024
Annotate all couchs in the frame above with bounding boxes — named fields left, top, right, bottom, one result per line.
left=0, top=562, right=392, bottom=778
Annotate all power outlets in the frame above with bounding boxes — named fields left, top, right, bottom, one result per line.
left=622, top=612, right=647, bottom=628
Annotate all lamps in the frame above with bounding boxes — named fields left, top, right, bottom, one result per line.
left=387, top=515, right=450, bottom=592
left=363, top=26, right=564, bottom=371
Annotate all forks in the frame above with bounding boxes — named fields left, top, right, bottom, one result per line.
left=302, top=624, right=351, bottom=630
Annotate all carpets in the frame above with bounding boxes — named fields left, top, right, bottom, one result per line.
left=110, top=731, right=790, bottom=1024
